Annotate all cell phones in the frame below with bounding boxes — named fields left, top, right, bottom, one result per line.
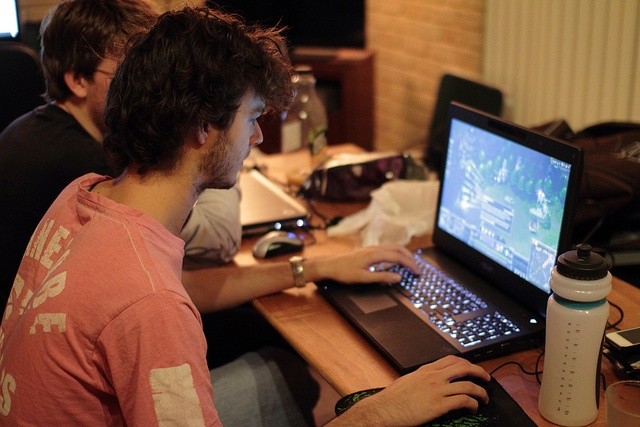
left=605, top=325, right=640, bottom=353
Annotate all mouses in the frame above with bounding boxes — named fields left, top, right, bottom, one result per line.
left=251, top=227, right=304, bottom=260
left=450, top=375, right=496, bottom=416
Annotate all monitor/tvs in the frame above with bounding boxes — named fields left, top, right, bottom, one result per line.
left=421, top=71, right=505, bottom=184
left=0, top=1, right=22, bottom=41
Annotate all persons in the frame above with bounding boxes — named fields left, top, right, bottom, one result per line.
left=1, top=0, right=244, bottom=322
left=1, top=7, right=493, bottom=426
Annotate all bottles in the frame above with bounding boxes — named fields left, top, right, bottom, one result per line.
left=281, top=65, right=328, bottom=181
left=538, top=243, right=614, bottom=427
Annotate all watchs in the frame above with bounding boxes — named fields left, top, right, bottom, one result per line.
left=289, top=254, right=307, bottom=289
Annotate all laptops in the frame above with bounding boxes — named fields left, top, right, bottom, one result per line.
left=311, top=97, right=586, bottom=377
left=233, top=168, right=313, bottom=239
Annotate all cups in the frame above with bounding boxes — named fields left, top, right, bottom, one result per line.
left=605, top=380, right=640, bottom=427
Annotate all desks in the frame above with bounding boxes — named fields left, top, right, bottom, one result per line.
left=207, top=142, right=636, bottom=427
